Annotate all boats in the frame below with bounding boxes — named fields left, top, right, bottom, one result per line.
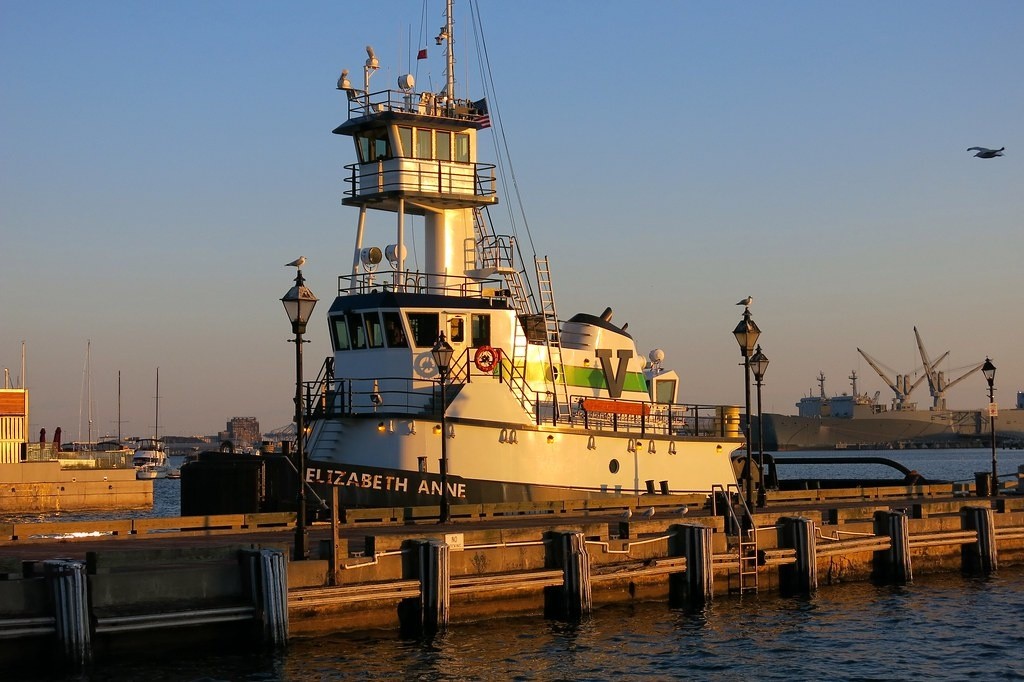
left=180, top=1, right=956, bottom=518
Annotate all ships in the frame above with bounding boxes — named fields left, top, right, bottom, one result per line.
left=733, top=326, right=1023, bottom=453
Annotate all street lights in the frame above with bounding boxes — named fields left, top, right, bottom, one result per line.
left=752, top=343, right=771, bottom=510
left=731, top=307, right=763, bottom=515
left=279, top=269, right=320, bottom=562
left=432, top=330, right=454, bottom=523
left=981, top=355, right=1000, bottom=496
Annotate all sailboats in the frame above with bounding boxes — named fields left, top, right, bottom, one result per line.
left=71, top=341, right=263, bottom=479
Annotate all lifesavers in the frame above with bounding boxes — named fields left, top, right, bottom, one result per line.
left=474, top=346, right=498, bottom=372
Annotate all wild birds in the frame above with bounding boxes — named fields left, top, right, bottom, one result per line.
left=735, top=295, right=753, bottom=307
left=967, top=147, right=1005, bottom=159
left=622, top=509, right=632, bottom=522
left=670, top=505, right=689, bottom=518
left=283, top=256, right=307, bottom=270
left=642, top=506, right=655, bottom=520
